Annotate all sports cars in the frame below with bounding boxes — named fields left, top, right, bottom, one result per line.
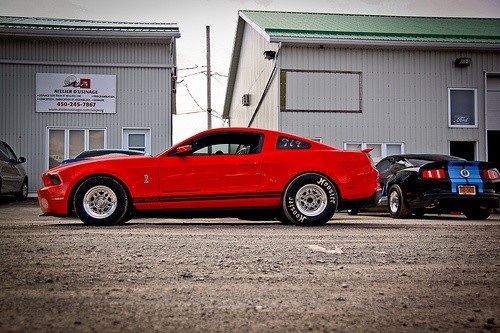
left=43, top=126, right=380, bottom=227
left=348, top=154, right=496, bottom=221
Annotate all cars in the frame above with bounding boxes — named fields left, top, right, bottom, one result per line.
left=1, top=140, right=29, bottom=203
left=58, top=148, right=150, bottom=167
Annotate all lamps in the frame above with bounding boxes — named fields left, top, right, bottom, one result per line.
left=454, top=57, right=471, bottom=67
